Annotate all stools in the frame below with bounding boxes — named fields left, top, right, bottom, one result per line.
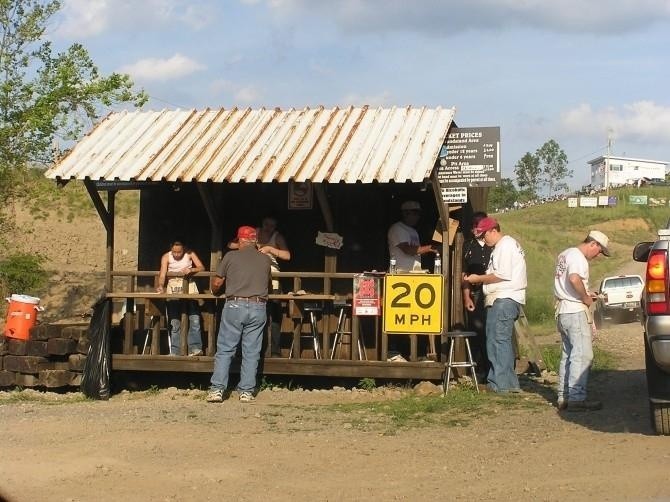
left=141, top=301, right=172, bottom=355
left=289, top=306, right=324, bottom=360
left=330, top=303, right=368, bottom=361
left=442, top=330, right=481, bottom=392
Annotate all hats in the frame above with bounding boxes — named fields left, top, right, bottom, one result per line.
left=401, top=201, right=422, bottom=211
left=588, top=230, right=611, bottom=257
left=475, top=217, right=498, bottom=238
left=233, top=226, right=257, bottom=240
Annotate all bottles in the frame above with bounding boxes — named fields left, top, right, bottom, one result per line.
left=390, top=254, right=396, bottom=274
left=434, top=253, right=442, bottom=275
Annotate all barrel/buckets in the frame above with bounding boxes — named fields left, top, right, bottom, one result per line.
left=3, top=293, right=43, bottom=343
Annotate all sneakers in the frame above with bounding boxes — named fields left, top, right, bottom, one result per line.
left=387, top=355, right=408, bottom=362
left=239, top=392, right=256, bottom=402
left=567, top=400, right=601, bottom=412
left=206, top=390, right=223, bottom=402
left=188, top=350, right=203, bottom=356
left=558, top=401, right=567, bottom=409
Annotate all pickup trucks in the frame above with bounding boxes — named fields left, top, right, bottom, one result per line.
left=642, top=176, right=670, bottom=188
left=631, top=218, right=670, bottom=435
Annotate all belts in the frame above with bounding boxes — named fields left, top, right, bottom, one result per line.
left=227, top=296, right=265, bottom=302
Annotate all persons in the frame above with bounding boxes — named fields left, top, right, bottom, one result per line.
left=466, top=218, right=526, bottom=387
left=227, top=211, right=291, bottom=357
left=157, top=238, right=206, bottom=355
left=463, top=211, right=500, bottom=381
left=499, top=177, right=644, bottom=213
left=553, top=231, right=612, bottom=411
left=207, top=225, right=271, bottom=402
left=386, top=199, right=437, bottom=363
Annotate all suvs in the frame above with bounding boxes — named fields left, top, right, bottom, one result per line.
left=576, top=185, right=590, bottom=196
left=593, top=275, right=646, bottom=327
